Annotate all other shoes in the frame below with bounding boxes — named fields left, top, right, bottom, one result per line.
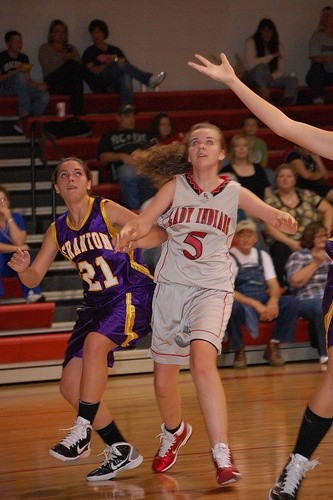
left=263, top=341, right=286, bottom=367
left=320, top=356, right=328, bottom=364
left=90, top=80, right=111, bottom=92
left=234, top=347, right=247, bottom=369
left=14, top=121, right=25, bottom=132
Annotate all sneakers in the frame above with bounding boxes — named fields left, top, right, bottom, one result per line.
left=121, top=103, right=135, bottom=114
left=152, top=421, right=192, bottom=473
left=148, top=71, right=166, bottom=89
left=268, top=453, right=320, bottom=500
left=211, top=443, right=241, bottom=486
left=49, top=416, right=92, bottom=461
left=87, top=443, right=144, bottom=481
left=26, top=293, right=46, bottom=303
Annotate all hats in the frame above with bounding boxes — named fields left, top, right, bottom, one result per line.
left=236, top=220, right=256, bottom=232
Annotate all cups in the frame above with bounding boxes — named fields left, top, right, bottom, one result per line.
left=57, top=102, right=65, bottom=117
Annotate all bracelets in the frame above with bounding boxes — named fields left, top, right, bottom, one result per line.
left=114, top=57, right=118, bottom=61
left=8, top=218, right=13, bottom=223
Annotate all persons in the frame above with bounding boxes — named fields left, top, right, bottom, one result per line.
left=187, top=54, right=333, bottom=500
left=7, top=156, right=170, bottom=483
left=117, top=121, right=299, bottom=486
left=0, top=19, right=333, bottom=367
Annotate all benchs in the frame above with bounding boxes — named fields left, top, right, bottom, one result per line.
left=0, top=85, right=333, bottom=366
